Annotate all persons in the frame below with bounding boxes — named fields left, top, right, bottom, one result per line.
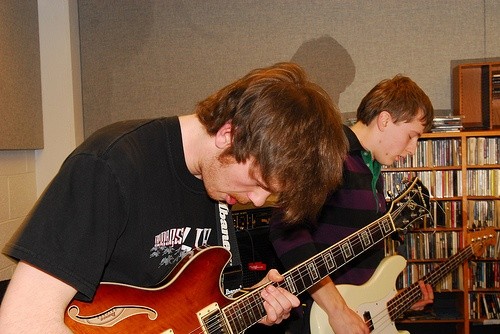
left=0, top=61, right=350, bottom=334
left=269, top=75, right=434, bottom=334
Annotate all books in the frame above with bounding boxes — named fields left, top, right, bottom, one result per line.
left=349, top=116, right=500, bottom=320
left=492, top=74, right=500, bottom=99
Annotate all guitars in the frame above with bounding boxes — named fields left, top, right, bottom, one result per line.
left=302, top=224, right=498, bottom=334
left=61, top=175, right=435, bottom=334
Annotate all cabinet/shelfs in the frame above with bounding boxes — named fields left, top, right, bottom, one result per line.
left=380, top=130, right=500, bottom=334
